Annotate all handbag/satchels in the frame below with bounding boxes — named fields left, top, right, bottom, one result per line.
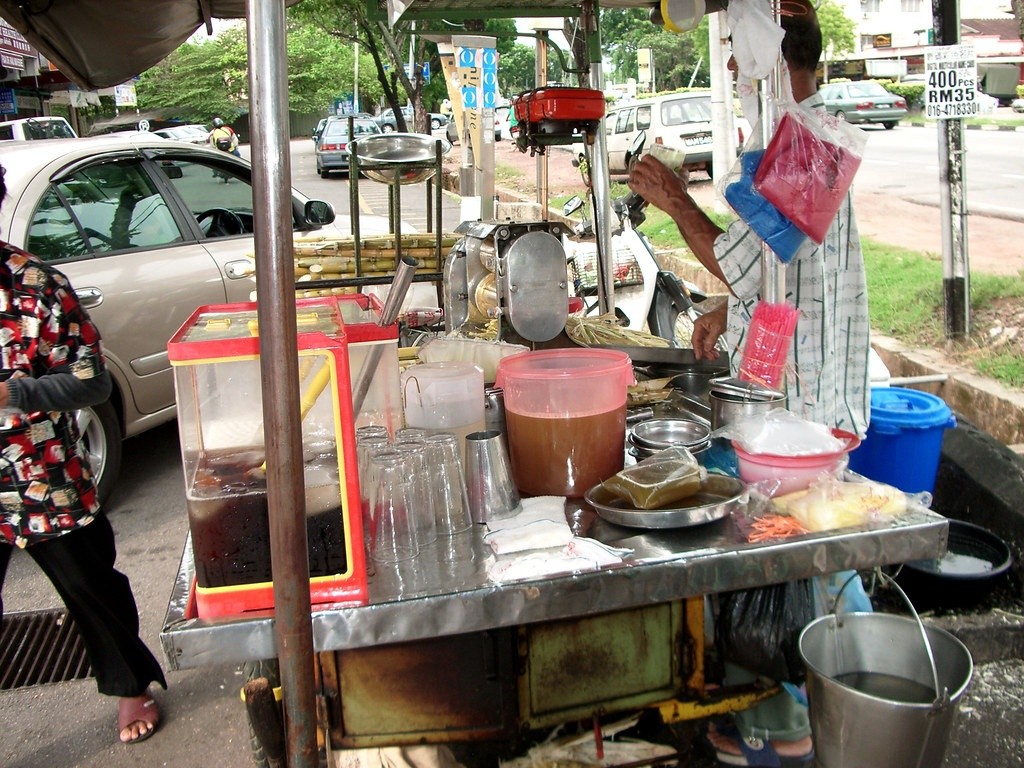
left=782, top=570, right=873, bottom=708
left=720, top=89, right=869, bottom=264
left=715, top=575, right=817, bottom=688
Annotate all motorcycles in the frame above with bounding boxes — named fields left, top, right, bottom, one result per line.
left=215, top=132, right=242, bottom=183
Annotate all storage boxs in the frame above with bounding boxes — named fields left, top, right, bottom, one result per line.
left=164, top=295, right=369, bottom=621
left=338, top=292, right=408, bottom=524
left=513, top=88, right=607, bottom=136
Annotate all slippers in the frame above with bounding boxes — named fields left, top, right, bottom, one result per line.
left=118, top=687, right=160, bottom=743
left=707, top=718, right=814, bottom=768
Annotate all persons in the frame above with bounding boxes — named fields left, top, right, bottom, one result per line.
left=626, top=0, right=872, bottom=614
left=0, top=163, right=164, bottom=745
left=210, top=119, right=240, bottom=175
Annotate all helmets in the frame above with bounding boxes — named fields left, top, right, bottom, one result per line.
left=212, top=118, right=224, bottom=128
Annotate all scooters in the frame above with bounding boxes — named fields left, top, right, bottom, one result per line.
left=507, top=120, right=520, bottom=142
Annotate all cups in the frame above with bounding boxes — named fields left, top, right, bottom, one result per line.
left=355, top=425, right=522, bottom=562
left=650, top=142, right=685, bottom=176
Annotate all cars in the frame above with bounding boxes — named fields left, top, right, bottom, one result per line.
left=818, top=80, right=908, bottom=129
left=312, top=118, right=382, bottom=178
left=0, top=137, right=440, bottom=512
left=371, top=107, right=448, bottom=133
left=152, top=125, right=211, bottom=146
left=312, top=111, right=374, bottom=143
left=445, top=109, right=501, bottom=145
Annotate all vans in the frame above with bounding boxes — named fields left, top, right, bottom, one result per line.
left=0, top=115, right=76, bottom=142
left=572, top=91, right=744, bottom=180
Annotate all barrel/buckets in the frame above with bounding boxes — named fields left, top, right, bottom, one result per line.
left=401, top=360, right=485, bottom=480
left=848, top=386, right=957, bottom=494
left=886, top=519, right=1011, bottom=617
left=494, top=348, right=635, bottom=499
left=799, top=569, right=973, bottom=768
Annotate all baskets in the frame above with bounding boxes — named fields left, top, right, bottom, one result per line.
left=574, top=244, right=644, bottom=295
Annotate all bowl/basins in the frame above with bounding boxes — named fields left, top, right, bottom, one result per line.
left=628, top=434, right=707, bottom=456
left=345, top=132, right=452, bottom=185
left=628, top=447, right=712, bottom=464
left=731, top=428, right=862, bottom=497
left=632, top=418, right=710, bottom=447
left=709, top=377, right=786, bottom=431
